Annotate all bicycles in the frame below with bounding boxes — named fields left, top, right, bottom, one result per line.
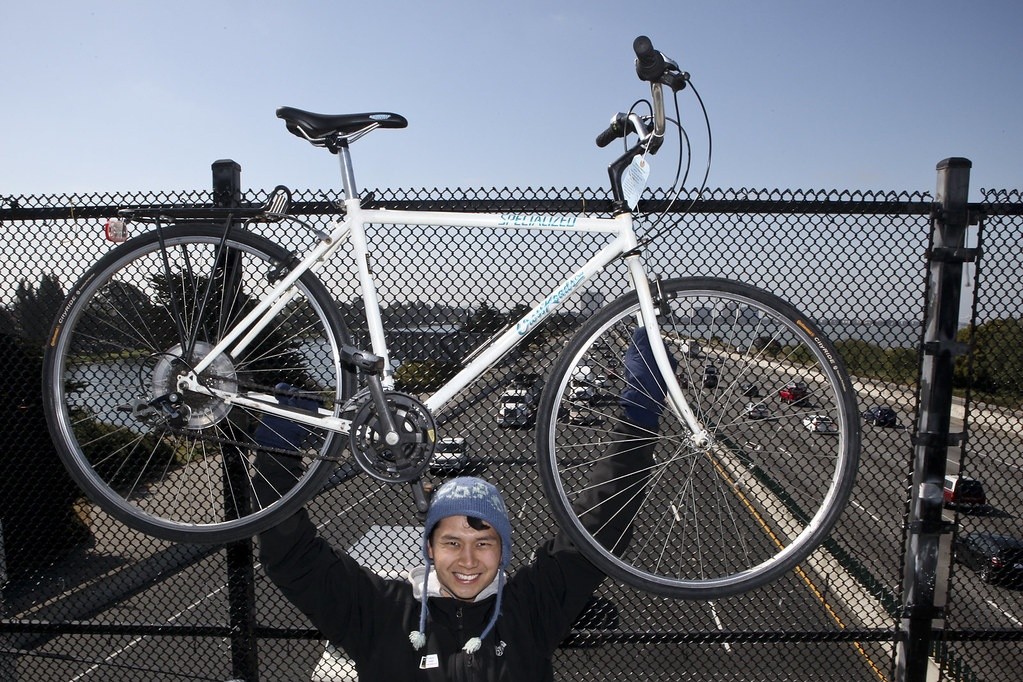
left=39, top=34, right=861, bottom=602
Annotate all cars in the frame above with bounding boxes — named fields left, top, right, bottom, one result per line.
left=604, top=351, right=612, bottom=358
left=803, top=415, right=834, bottom=434
left=740, top=384, right=759, bottom=396
left=595, top=376, right=607, bottom=387
left=743, top=402, right=769, bottom=419
left=676, top=374, right=688, bottom=387
left=788, top=383, right=807, bottom=393
left=705, top=365, right=718, bottom=374
left=716, top=357, right=726, bottom=364
left=608, top=359, right=617, bottom=367
left=622, top=356, right=625, bottom=366
left=608, top=369, right=617, bottom=378
left=955, top=533, right=1023, bottom=582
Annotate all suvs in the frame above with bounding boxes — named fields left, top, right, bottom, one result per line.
left=780, top=387, right=804, bottom=405
left=569, top=400, right=592, bottom=424
left=429, top=437, right=470, bottom=474
left=863, top=407, right=897, bottom=426
left=943, top=475, right=988, bottom=510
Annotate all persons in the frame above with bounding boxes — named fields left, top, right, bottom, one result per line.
left=248, top=318, right=681, bottom=682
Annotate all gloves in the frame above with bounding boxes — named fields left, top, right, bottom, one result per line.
left=621, top=325, right=677, bottom=427
left=254, top=382, right=319, bottom=450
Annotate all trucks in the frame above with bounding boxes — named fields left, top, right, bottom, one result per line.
left=569, top=366, right=597, bottom=405
left=496, top=372, right=541, bottom=427
left=309, top=526, right=424, bottom=682
left=591, top=341, right=599, bottom=349
left=680, top=339, right=699, bottom=357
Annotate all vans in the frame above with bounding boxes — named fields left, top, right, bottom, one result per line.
left=704, top=375, right=718, bottom=386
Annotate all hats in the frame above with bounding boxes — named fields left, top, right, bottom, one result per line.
left=409, top=477, right=511, bottom=656
